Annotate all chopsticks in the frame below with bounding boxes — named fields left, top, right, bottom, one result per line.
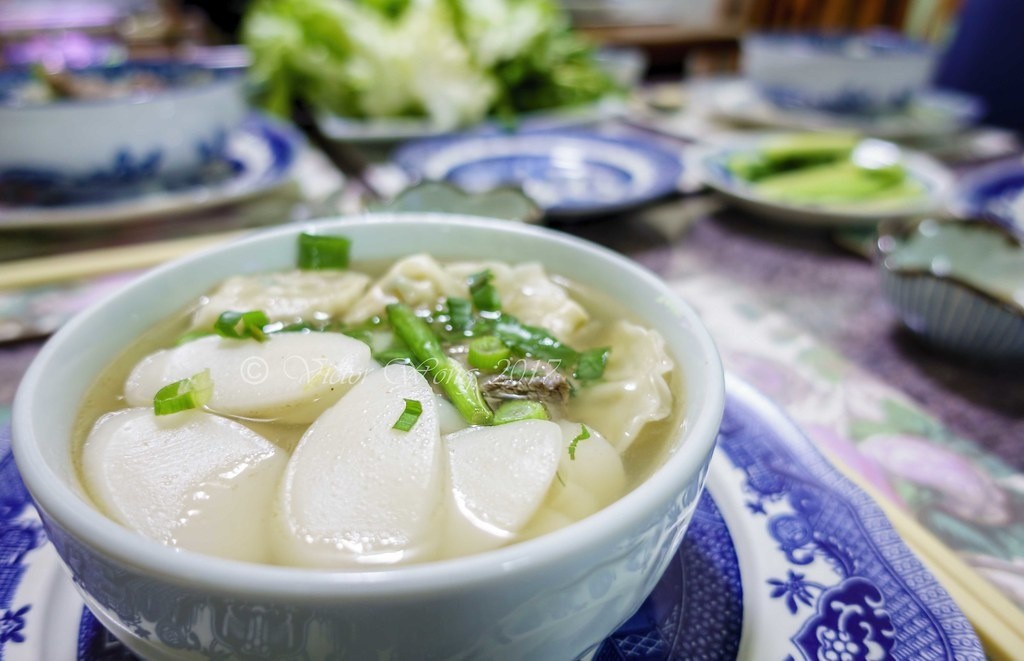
left=809, top=433, right=1024, bottom=661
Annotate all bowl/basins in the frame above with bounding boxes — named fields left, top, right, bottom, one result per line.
left=741, top=30, right=937, bottom=113
left=0, top=40, right=255, bottom=199
left=14, top=216, right=724, bottom=661
left=876, top=214, right=1024, bottom=377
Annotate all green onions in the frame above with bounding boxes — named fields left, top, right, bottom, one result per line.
left=147, top=236, right=615, bottom=458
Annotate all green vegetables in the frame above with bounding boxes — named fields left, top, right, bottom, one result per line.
left=240, top=0, right=638, bottom=130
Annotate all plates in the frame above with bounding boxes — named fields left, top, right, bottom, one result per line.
left=0, top=113, right=305, bottom=224
left=391, top=125, right=684, bottom=216
left=695, top=75, right=985, bottom=138
left=957, top=152, right=1024, bottom=246
left=249, top=184, right=540, bottom=230
left=692, top=130, right=955, bottom=233
left=311, top=52, right=644, bottom=138
left=0, top=367, right=982, bottom=661
